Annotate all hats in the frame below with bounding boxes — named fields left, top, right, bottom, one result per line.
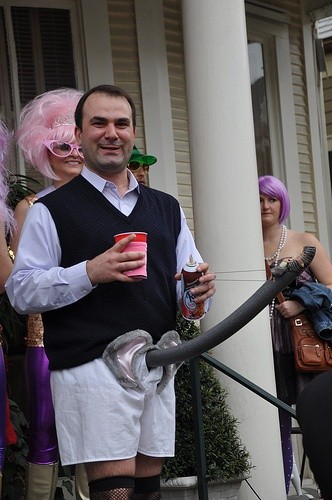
left=127, top=144, right=157, bottom=166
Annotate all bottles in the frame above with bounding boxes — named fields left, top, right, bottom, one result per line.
left=180, top=254, right=207, bottom=321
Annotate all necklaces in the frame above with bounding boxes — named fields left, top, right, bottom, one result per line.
left=264, top=225, right=287, bottom=325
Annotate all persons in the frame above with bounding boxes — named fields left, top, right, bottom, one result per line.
left=0, top=85, right=216, bottom=500
left=258, top=175, right=332, bottom=500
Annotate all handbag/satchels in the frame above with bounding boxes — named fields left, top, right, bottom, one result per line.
left=290, top=313, right=332, bottom=371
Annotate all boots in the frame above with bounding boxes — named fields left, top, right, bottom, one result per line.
left=24, top=462, right=58, bottom=500
left=74, top=463, right=90, bottom=500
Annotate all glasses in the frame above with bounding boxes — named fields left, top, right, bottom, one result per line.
left=43, top=140, right=84, bottom=159
left=128, top=161, right=149, bottom=173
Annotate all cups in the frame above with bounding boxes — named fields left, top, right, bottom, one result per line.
left=114, top=232, right=148, bottom=279
